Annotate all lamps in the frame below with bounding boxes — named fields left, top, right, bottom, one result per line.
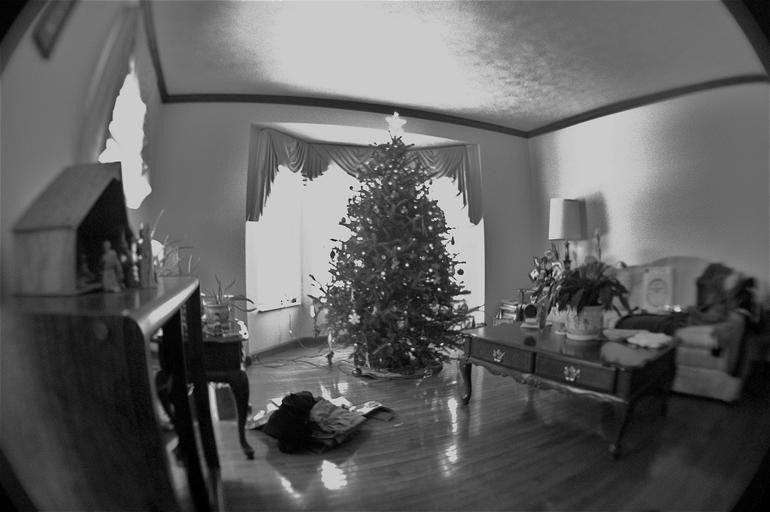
left=548, top=198, right=584, bottom=271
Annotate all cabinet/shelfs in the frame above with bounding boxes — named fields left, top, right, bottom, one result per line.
left=1, top=277, right=226, bottom=512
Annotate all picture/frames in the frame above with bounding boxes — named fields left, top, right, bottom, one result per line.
left=32, top=0, right=76, bottom=58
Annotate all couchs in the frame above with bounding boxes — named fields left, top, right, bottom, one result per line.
left=523, top=258, right=762, bottom=405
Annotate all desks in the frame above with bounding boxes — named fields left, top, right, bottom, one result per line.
left=162, top=317, right=257, bottom=463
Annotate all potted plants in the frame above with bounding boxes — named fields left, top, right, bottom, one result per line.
left=311, top=140, right=482, bottom=378
left=204, top=274, right=257, bottom=335
left=556, top=263, right=630, bottom=341
